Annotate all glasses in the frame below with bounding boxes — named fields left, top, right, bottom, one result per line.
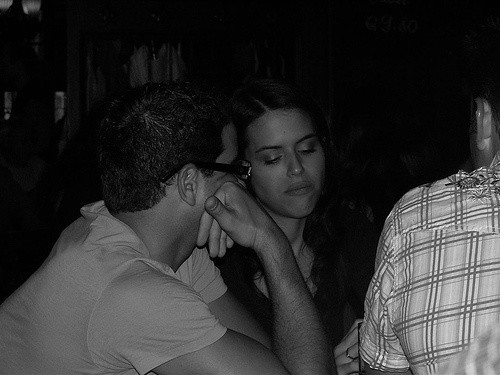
left=175, top=155, right=252, bottom=180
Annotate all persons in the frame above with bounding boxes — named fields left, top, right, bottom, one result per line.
left=0, top=80, right=337, bottom=375
left=210, top=78, right=381, bottom=375
left=359, top=71, right=499, bottom=374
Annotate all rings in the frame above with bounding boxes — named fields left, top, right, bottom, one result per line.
left=346, top=348, right=354, bottom=361
